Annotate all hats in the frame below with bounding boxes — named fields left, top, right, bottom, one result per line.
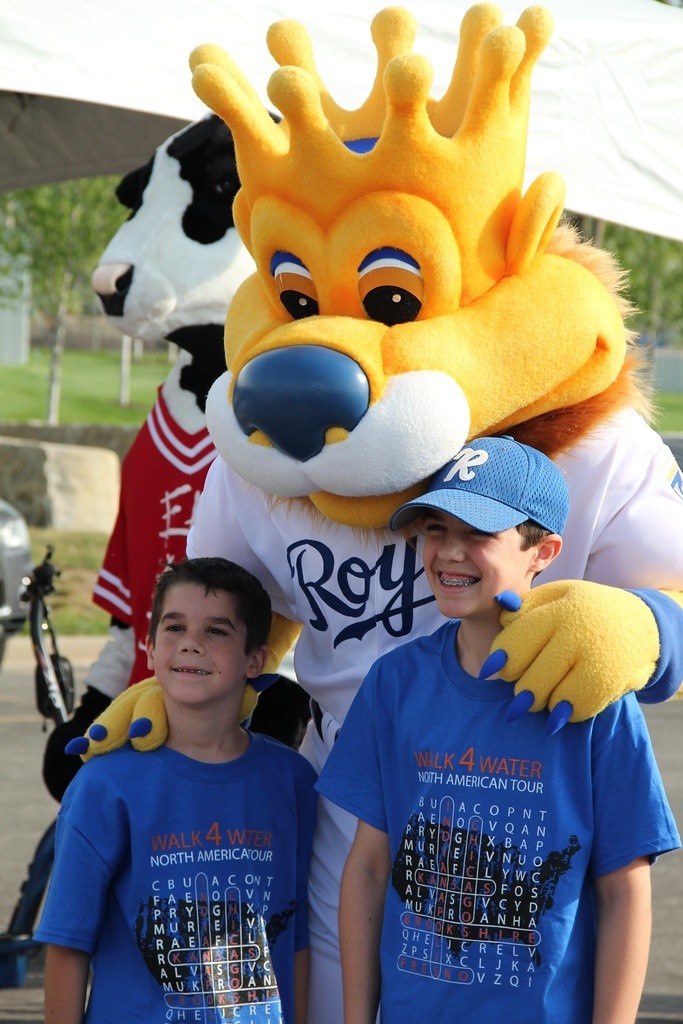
left=391, top=435, right=568, bottom=533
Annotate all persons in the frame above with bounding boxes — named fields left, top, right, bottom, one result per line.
left=30, top=558, right=322, bottom=1024
left=312, top=436, right=683, bottom=1024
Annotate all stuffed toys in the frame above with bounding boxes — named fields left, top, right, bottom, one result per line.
left=39, top=109, right=284, bottom=803
left=64, top=0, right=682, bottom=1024
